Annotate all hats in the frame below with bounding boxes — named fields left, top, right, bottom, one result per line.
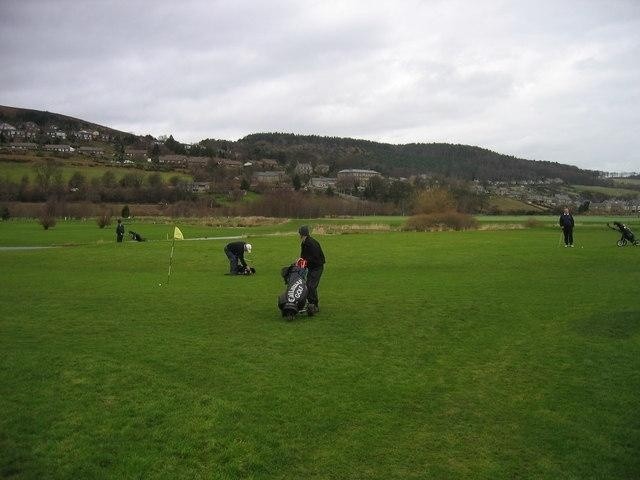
left=247, top=244, right=252, bottom=253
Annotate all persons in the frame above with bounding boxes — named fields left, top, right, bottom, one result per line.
left=116, top=219, right=124, bottom=242
left=298, top=226, right=326, bottom=311
left=559, top=207, right=574, bottom=248
left=223, top=241, right=252, bottom=277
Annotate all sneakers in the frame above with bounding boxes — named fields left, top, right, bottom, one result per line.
left=308, top=303, right=318, bottom=315
left=564, top=244, right=574, bottom=248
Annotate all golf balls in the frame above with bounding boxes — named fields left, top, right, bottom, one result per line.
left=159, top=284, right=162, bottom=286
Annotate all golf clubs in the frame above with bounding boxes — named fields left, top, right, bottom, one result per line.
left=573, top=229, right=583, bottom=249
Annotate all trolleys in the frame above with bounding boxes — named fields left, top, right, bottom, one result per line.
left=282, top=259, right=316, bottom=322
left=607, top=222, right=640, bottom=247
